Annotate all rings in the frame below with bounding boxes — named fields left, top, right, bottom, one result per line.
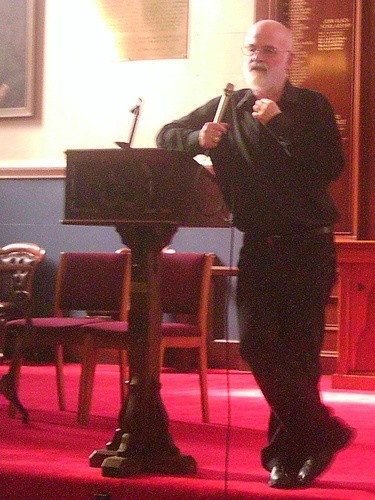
left=255, top=105, right=261, bottom=112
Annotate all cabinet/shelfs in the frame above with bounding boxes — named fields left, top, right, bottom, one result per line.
left=253, top=0, right=375, bottom=392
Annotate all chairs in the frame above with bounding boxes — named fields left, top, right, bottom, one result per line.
left=0, top=243, right=239, bottom=424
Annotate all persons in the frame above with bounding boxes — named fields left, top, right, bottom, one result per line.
left=155, top=19, right=355, bottom=488
left=0, top=35, right=25, bottom=108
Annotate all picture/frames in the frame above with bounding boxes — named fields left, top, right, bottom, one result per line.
left=0, top=0, right=37, bottom=120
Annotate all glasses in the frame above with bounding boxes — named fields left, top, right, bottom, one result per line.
left=241, top=46, right=291, bottom=58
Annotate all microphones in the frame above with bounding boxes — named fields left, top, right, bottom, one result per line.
left=205, top=83, right=234, bottom=155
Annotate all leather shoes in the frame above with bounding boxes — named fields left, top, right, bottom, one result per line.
left=298, top=418, right=355, bottom=488
left=268, top=464, right=301, bottom=489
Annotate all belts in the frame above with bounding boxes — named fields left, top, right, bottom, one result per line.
left=243, top=226, right=332, bottom=241
